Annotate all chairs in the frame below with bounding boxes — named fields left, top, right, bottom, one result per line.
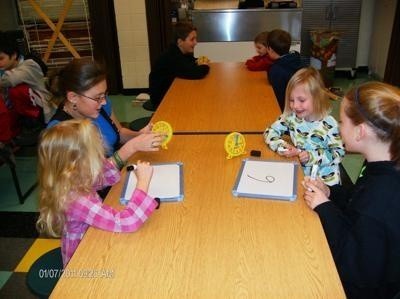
left=8, top=84, right=41, bottom=119
left=0, top=94, right=24, bottom=204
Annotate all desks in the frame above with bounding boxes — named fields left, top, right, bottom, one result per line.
left=48, top=134, right=347, bottom=299
left=148, top=41, right=283, bottom=134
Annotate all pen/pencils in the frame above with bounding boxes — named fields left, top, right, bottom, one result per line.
left=127, top=165, right=137, bottom=171
left=308, top=164, right=319, bottom=192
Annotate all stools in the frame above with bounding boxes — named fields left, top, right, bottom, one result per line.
left=26, top=248, right=63, bottom=299
left=130, top=117, right=151, bottom=131
left=143, top=99, right=158, bottom=111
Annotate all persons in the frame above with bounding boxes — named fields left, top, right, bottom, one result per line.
left=149, top=21, right=209, bottom=106
left=267, top=29, right=304, bottom=111
left=43, top=57, right=161, bottom=170
left=246, top=31, right=272, bottom=71
left=36, top=118, right=153, bottom=267
left=304, top=82, right=400, bottom=299
left=263, top=67, right=346, bottom=189
left=0, top=37, right=54, bottom=165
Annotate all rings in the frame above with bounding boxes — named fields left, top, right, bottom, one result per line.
left=151, top=142, right=155, bottom=147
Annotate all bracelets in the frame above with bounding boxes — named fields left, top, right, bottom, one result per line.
left=113, top=151, right=124, bottom=169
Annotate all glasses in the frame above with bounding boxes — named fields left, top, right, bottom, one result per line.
left=74, top=90, right=109, bottom=104
